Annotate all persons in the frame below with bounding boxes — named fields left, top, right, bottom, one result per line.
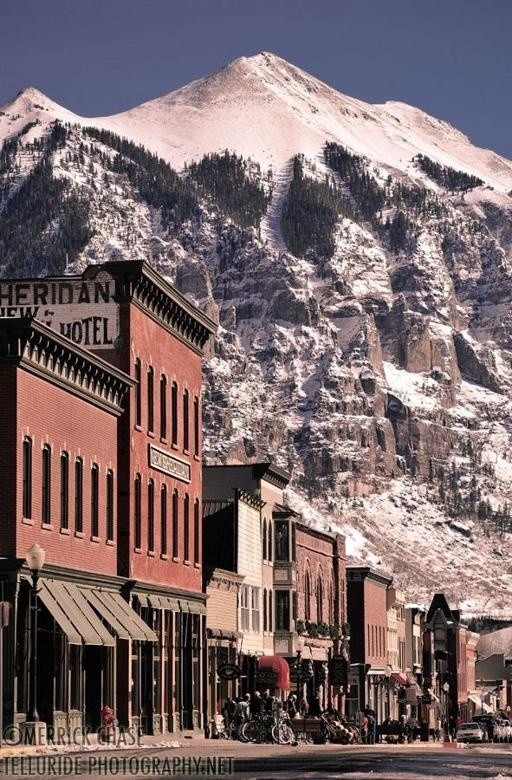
left=486, top=717, right=511, bottom=744
left=434, top=715, right=463, bottom=742
left=398, top=714, right=408, bottom=744
left=325, top=702, right=337, bottom=717
left=407, top=713, right=417, bottom=744
left=223, top=689, right=308, bottom=740
left=364, top=705, right=376, bottom=716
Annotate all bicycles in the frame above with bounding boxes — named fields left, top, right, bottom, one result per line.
left=236, top=706, right=296, bottom=745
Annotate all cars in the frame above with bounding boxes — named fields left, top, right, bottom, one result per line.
left=455, top=715, right=512, bottom=743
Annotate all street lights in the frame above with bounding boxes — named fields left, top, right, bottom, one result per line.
left=385, top=666, right=392, bottom=723
left=24, top=542, right=45, bottom=728
left=443, top=682, right=450, bottom=734
